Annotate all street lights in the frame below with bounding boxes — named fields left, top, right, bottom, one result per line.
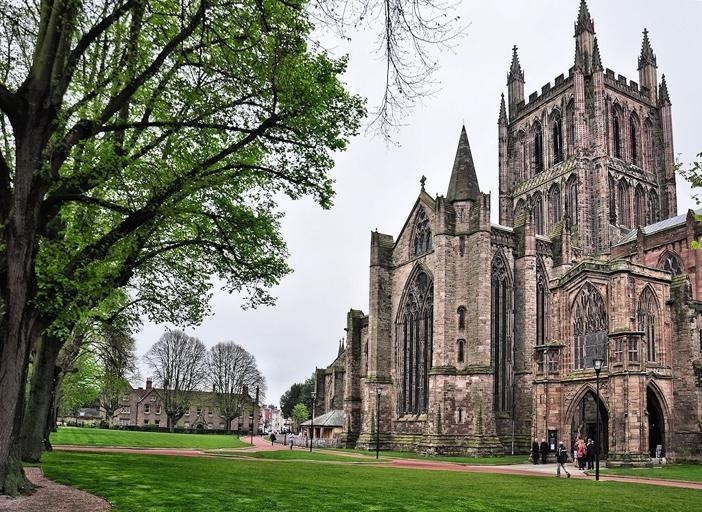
left=592, top=358, right=603, bottom=481
left=310, top=392, right=317, bottom=452
left=251, top=396, right=256, bottom=444
left=376, top=387, right=383, bottom=459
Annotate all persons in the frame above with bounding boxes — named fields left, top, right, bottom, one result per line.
left=532, top=434, right=598, bottom=479
left=266, top=430, right=296, bottom=451
